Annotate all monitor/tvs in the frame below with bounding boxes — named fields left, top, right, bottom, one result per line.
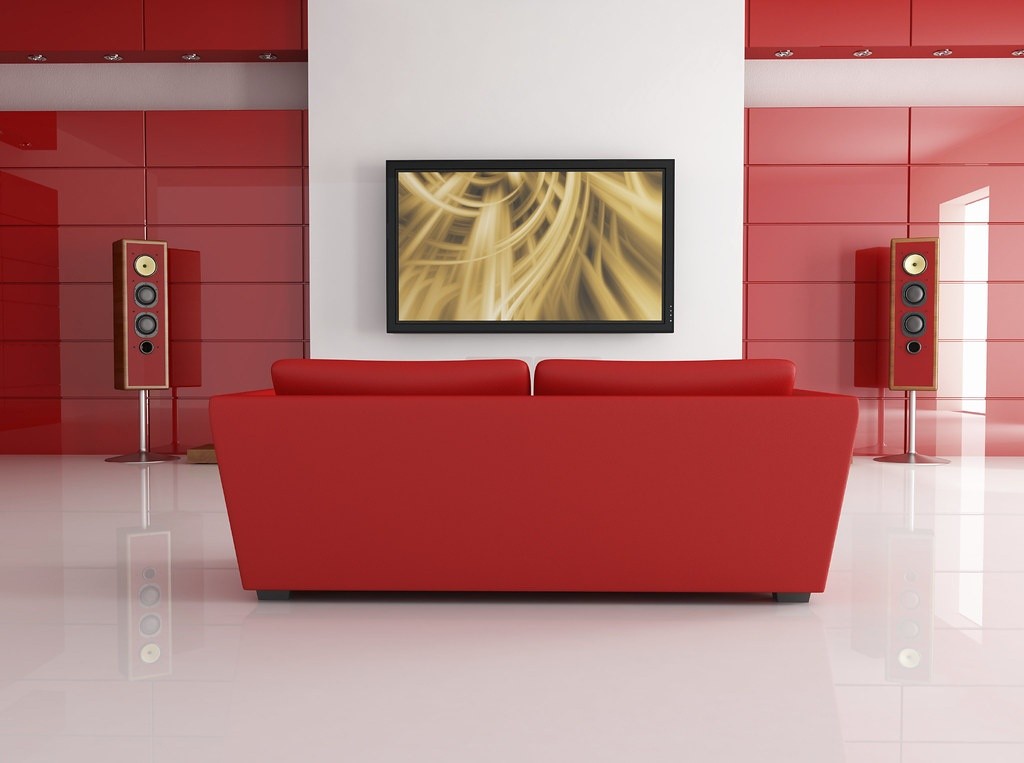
left=385, top=159, right=676, bottom=333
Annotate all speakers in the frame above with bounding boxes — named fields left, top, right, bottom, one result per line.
left=112, top=238, right=170, bottom=390
left=854, top=248, right=892, bottom=388
left=889, top=236, right=939, bottom=390
left=167, top=248, right=202, bottom=386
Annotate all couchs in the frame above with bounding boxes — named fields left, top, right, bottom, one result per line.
left=210, top=359, right=859, bottom=601
